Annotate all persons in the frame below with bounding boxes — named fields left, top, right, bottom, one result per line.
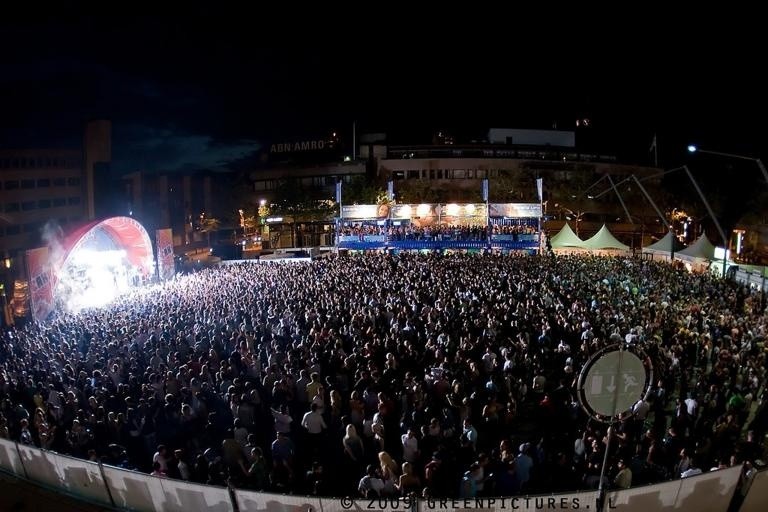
left=377, top=203, right=391, bottom=218
left=338, top=223, right=537, bottom=243
left=1, top=248, right=767, bottom=501
